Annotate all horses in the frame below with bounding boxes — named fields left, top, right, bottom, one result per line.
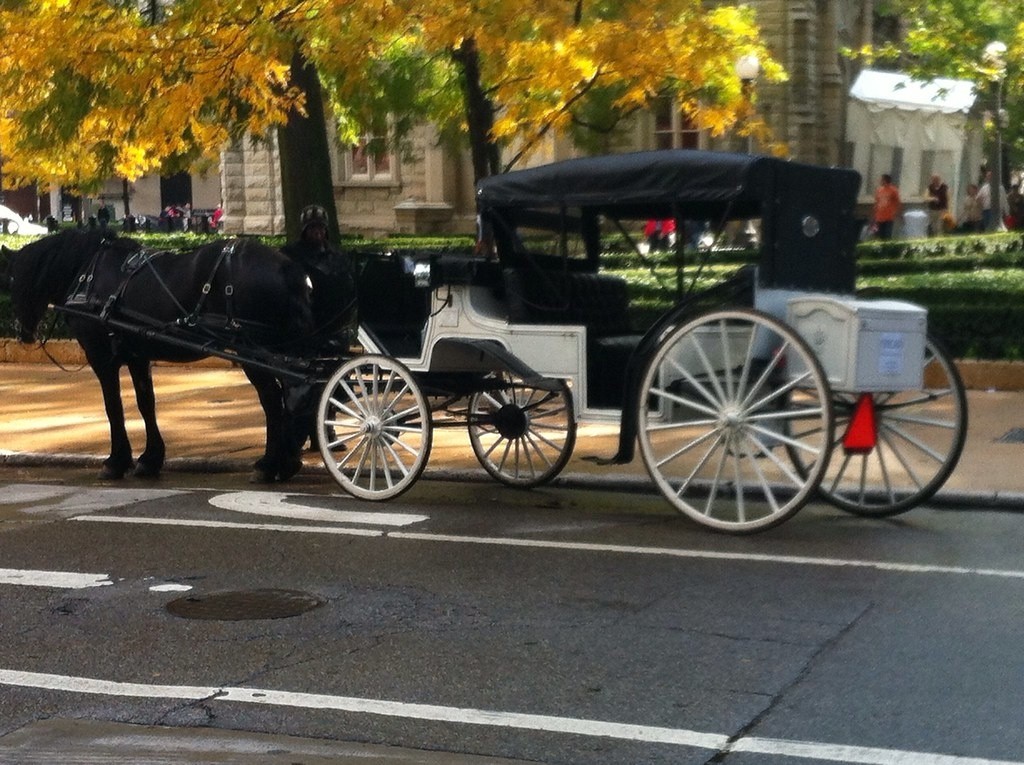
left=0, top=222, right=374, bottom=486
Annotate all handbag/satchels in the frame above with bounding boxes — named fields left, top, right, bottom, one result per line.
left=860, top=223, right=879, bottom=243
left=943, top=214, right=957, bottom=233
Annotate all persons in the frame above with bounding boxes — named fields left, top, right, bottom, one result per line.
left=964, top=184, right=982, bottom=233
left=645, top=220, right=675, bottom=252
left=166, top=202, right=190, bottom=232
left=97, top=199, right=110, bottom=223
left=976, top=170, right=1009, bottom=233
left=213, top=204, right=221, bottom=223
left=1007, top=185, right=1023, bottom=217
left=925, top=175, right=951, bottom=237
left=874, top=174, right=900, bottom=241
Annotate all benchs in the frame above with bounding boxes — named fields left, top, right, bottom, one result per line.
left=501, top=269, right=645, bottom=355
left=430, top=209, right=491, bottom=284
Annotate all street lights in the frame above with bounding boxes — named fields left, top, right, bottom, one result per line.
left=734, top=54, right=757, bottom=154
left=983, top=40, right=1008, bottom=233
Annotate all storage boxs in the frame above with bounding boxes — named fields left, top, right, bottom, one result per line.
left=784, top=293, right=927, bottom=393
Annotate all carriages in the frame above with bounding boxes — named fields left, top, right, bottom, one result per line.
left=0, top=149, right=969, bottom=535
